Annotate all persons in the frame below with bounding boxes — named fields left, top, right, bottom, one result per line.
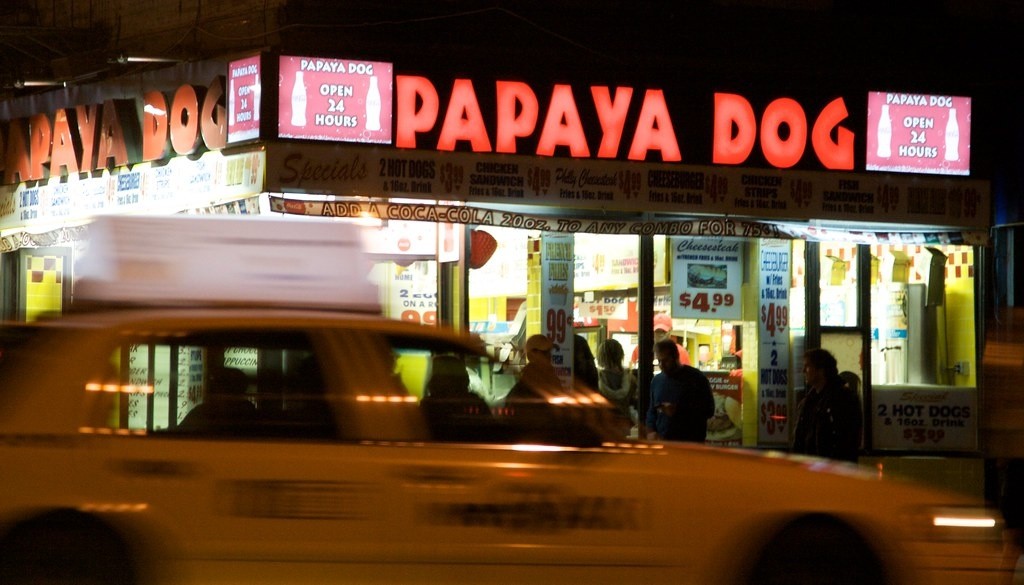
left=730, top=510, right=898, bottom=585
left=597, top=339, right=640, bottom=429
left=645, top=340, right=715, bottom=444
left=791, top=350, right=864, bottom=465
left=629, top=319, right=690, bottom=369
left=0, top=505, right=149, bottom=585
left=505, top=334, right=564, bottom=410
left=574, top=335, right=600, bottom=390
left=422, top=357, right=496, bottom=425
left=178, top=355, right=333, bottom=426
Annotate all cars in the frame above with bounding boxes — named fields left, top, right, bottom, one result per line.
left=1, top=209, right=1018, bottom=583
